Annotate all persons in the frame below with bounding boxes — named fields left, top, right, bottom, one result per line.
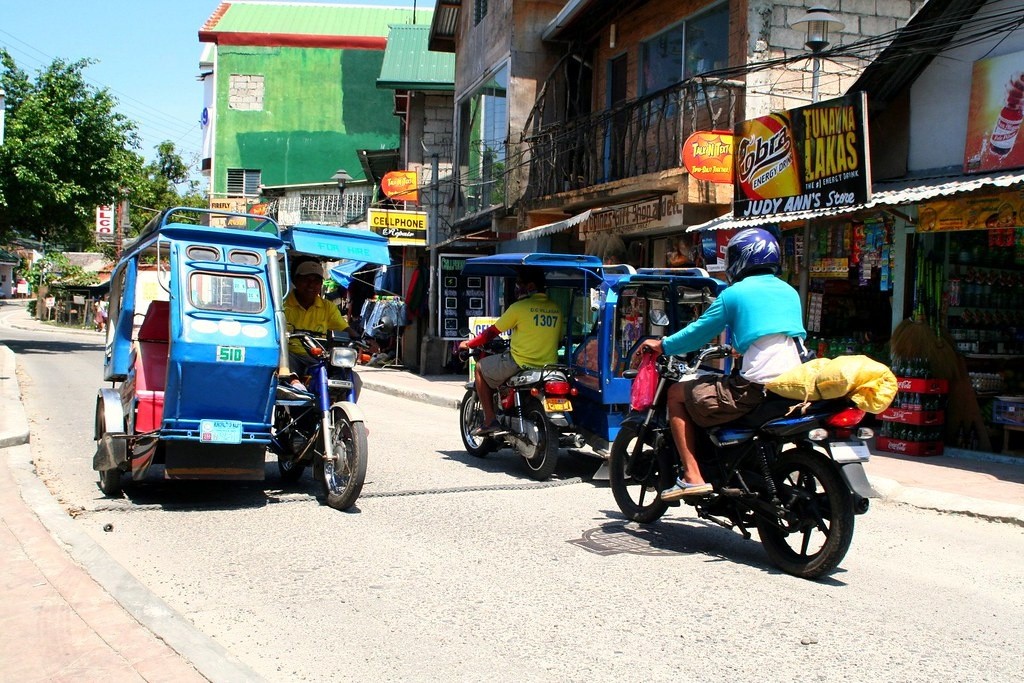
left=280, top=260, right=381, bottom=404
left=93, top=295, right=107, bottom=332
left=636, top=227, right=806, bottom=502
left=457, top=269, right=565, bottom=437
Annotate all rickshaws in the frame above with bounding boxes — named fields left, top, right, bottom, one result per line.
left=93, top=206, right=392, bottom=510
left=459, top=252, right=728, bottom=481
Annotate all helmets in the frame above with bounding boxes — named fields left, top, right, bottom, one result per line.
left=724, top=228, right=780, bottom=286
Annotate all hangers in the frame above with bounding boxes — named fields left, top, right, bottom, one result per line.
left=365, top=294, right=401, bottom=305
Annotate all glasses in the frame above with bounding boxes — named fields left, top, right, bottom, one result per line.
left=515, top=280, right=523, bottom=288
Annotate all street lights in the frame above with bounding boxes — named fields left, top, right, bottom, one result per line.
left=763, top=5, right=845, bottom=330
left=331, top=168, right=356, bottom=266
left=420, top=136, right=448, bottom=375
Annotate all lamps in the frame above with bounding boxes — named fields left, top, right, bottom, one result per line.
left=609, top=23, right=616, bottom=48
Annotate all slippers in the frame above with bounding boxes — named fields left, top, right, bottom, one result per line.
left=661, top=477, right=714, bottom=501
left=472, top=424, right=502, bottom=437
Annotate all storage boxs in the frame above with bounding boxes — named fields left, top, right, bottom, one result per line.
left=991, top=401, right=1024, bottom=426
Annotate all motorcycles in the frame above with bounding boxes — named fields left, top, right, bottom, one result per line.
left=608, top=343, right=877, bottom=579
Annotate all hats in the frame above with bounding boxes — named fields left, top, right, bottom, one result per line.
left=294, top=262, right=325, bottom=280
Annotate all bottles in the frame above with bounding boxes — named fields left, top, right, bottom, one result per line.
left=809, top=336, right=855, bottom=360
left=880, top=355, right=942, bottom=442
left=947, top=275, right=1016, bottom=353
left=990, top=74, right=1024, bottom=155
left=968, top=371, right=1003, bottom=393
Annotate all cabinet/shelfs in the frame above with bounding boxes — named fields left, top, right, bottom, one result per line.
left=873, top=376, right=947, bottom=456
left=931, top=259, right=1024, bottom=397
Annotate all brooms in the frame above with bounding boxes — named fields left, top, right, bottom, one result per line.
left=891, top=232, right=936, bottom=361
left=924, top=232, right=966, bottom=379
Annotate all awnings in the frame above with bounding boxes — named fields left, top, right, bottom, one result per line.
left=517, top=196, right=660, bottom=242
left=685, top=168, right=1023, bottom=232
